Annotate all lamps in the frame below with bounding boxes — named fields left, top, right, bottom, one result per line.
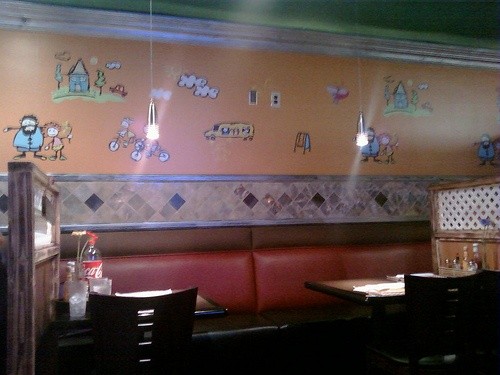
left=355, top=0, right=368, bottom=146
left=145, top=0, right=159, bottom=141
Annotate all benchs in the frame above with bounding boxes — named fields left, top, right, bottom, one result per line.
left=55, top=220, right=434, bottom=375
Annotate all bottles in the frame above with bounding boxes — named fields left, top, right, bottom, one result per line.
left=81, top=240, right=102, bottom=283
left=444, top=243, right=483, bottom=270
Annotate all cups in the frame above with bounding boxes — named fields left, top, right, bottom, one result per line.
left=90, top=277, right=112, bottom=296
left=66, top=279, right=88, bottom=316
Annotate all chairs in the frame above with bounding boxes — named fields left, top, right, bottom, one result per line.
left=90, top=287, right=198, bottom=375
left=373, top=269, right=500, bottom=375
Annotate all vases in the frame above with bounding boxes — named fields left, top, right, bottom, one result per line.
left=67, top=280, right=86, bottom=320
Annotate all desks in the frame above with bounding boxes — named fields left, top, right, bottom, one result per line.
left=53, top=297, right=230, bottom=330
left=305, top=272, right=462, bottom=343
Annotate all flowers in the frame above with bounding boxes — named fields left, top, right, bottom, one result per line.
left=71, top=230, right=97, bottom=280
